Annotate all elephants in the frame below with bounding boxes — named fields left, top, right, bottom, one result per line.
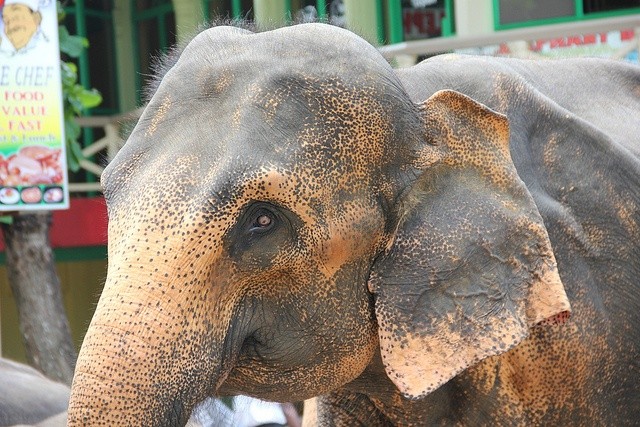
left=67, top=6, right=639, bottom=427
